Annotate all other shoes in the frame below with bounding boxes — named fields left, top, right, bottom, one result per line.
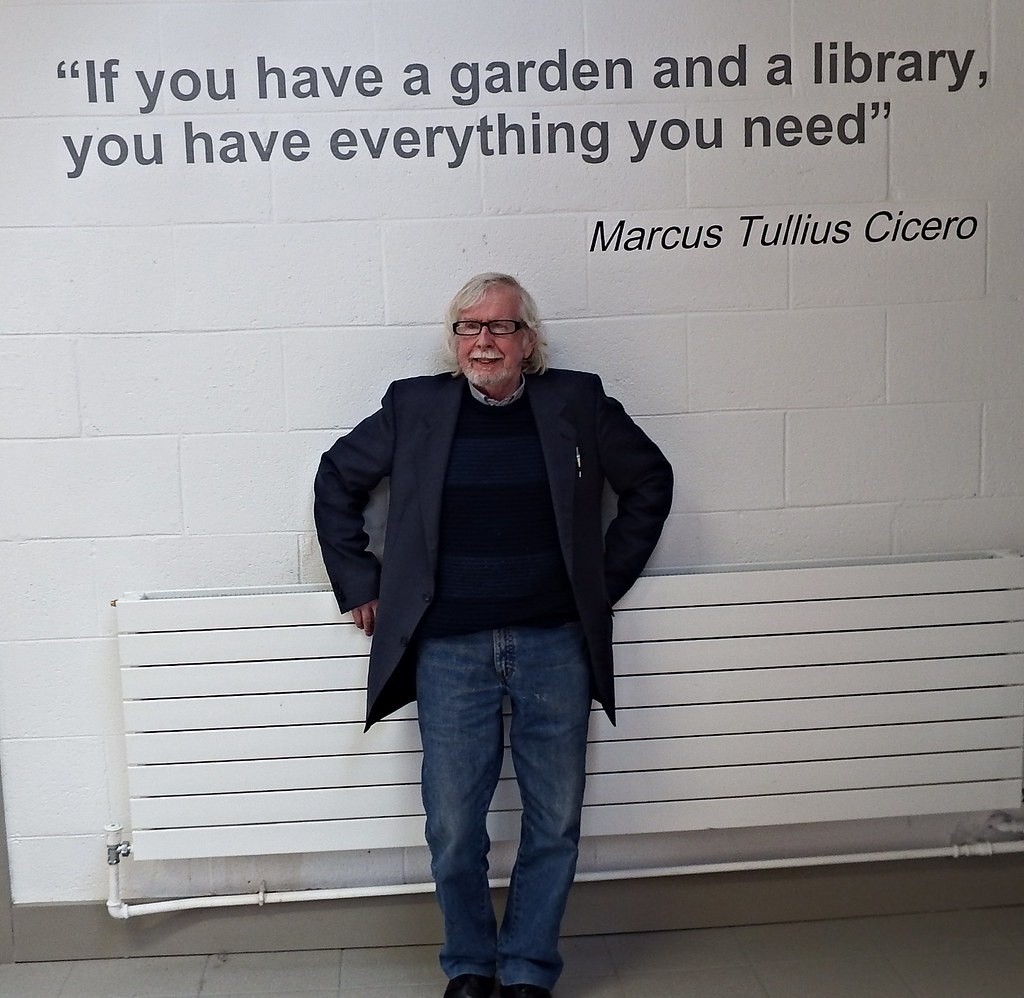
left=499, top=983, right=551, bottom=998
left=443, top=974, right=495, bottom=998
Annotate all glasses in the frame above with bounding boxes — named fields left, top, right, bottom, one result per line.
left=453, top=320, right=527, bottom=335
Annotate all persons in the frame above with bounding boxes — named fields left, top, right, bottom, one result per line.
left=310, top=274, right=676, bottom=998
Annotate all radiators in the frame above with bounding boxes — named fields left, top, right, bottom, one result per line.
left=104, top=552, right=1024, bottom=919
